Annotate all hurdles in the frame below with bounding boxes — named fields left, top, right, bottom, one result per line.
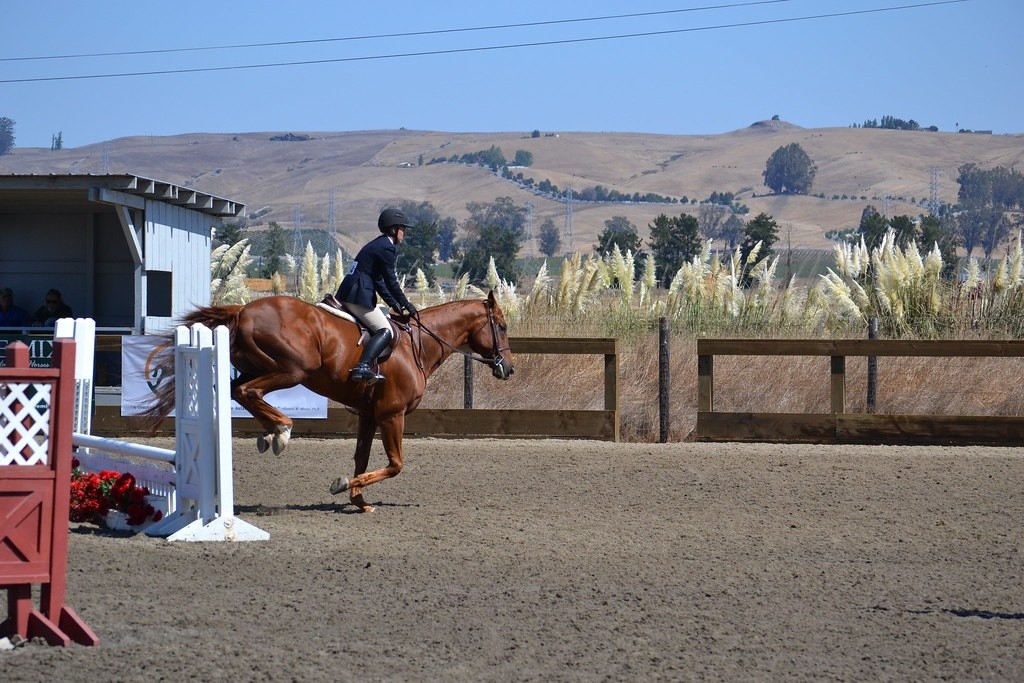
left=55, top=313, right=269, bottom=544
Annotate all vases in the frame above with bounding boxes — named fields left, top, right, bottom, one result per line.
left=106, top=509, right=132, bottom=530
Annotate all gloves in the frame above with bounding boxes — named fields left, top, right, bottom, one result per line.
left=406, top=302, right=416, bottom=314
left=392, top=304, right=403, bottom=314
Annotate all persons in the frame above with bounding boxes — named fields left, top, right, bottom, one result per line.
left=0, top=287, right=31, bottom=327
left=334, top=208, right=418, bottom=385
left=32, top=289, right=72, bottom=327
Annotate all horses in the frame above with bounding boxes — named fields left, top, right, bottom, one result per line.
left=125, top=288, right=517, bottom=513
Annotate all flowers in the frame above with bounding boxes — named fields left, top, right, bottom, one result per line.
left=69, top=456, right=162, bottom=526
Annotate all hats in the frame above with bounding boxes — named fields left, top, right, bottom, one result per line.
left=378, top=209, right=415, bottom=231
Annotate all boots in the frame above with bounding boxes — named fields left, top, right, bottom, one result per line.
left=350, top=328, right=392, bottom=384
